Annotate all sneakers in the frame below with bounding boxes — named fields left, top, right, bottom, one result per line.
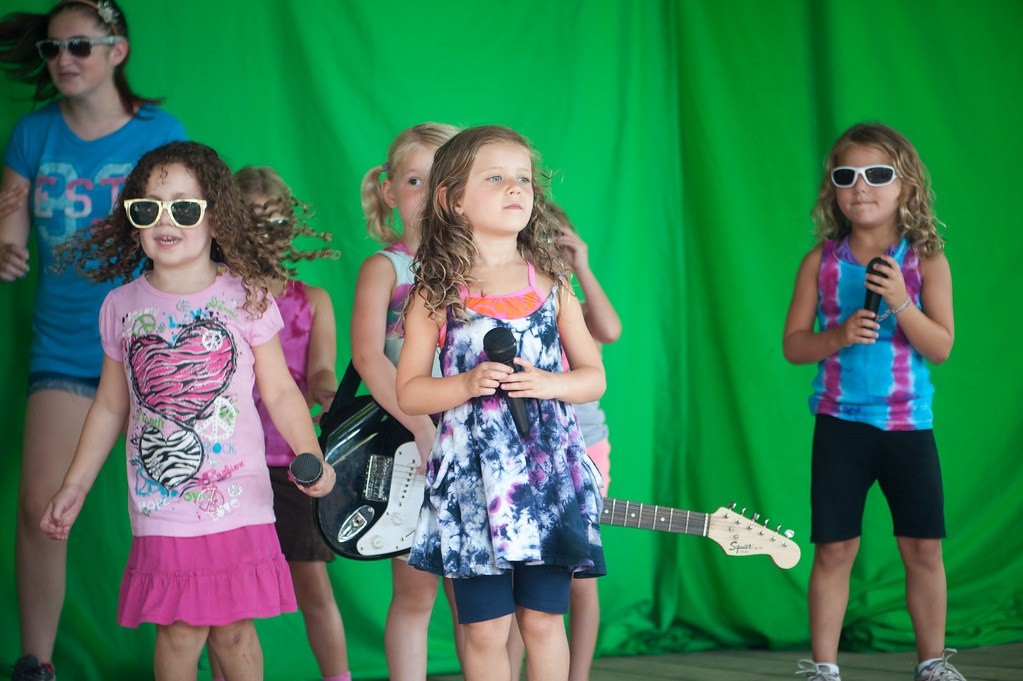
left=914, top=648, right=967, bottom=681
left=795, top=659, right=841, bottom=681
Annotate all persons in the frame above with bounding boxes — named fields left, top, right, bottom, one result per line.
left=0, top=0, right=191, bottom=681
left=0, top=183, right=28, bottom=220
left=38, top=140, right=351, bottom=681
left=781, top=121, right=967, bottom=681
left=351, top=123, right=622, bottom=681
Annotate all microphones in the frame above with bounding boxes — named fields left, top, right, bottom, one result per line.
left=483, top=327, right=531, bottom=441
left=288, top=454, right=322, bottom=488
left=862, top=258, right=890, bottom=339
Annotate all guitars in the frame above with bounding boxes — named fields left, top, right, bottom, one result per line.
left=309, top=391, right=803, bottom=573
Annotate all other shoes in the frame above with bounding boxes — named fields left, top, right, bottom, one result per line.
left=10, top=655, right=55, bottom=681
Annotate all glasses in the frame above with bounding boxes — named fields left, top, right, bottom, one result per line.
left=36, top=36, right=126, bottom=63
left=254, top=217, right=292, bottom=243
left=124, top=198, right=210, bottom=229
left=830, top=164, right=903, bottom=188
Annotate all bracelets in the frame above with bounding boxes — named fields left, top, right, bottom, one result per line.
left=877, top=293, right=913, bottom=322
